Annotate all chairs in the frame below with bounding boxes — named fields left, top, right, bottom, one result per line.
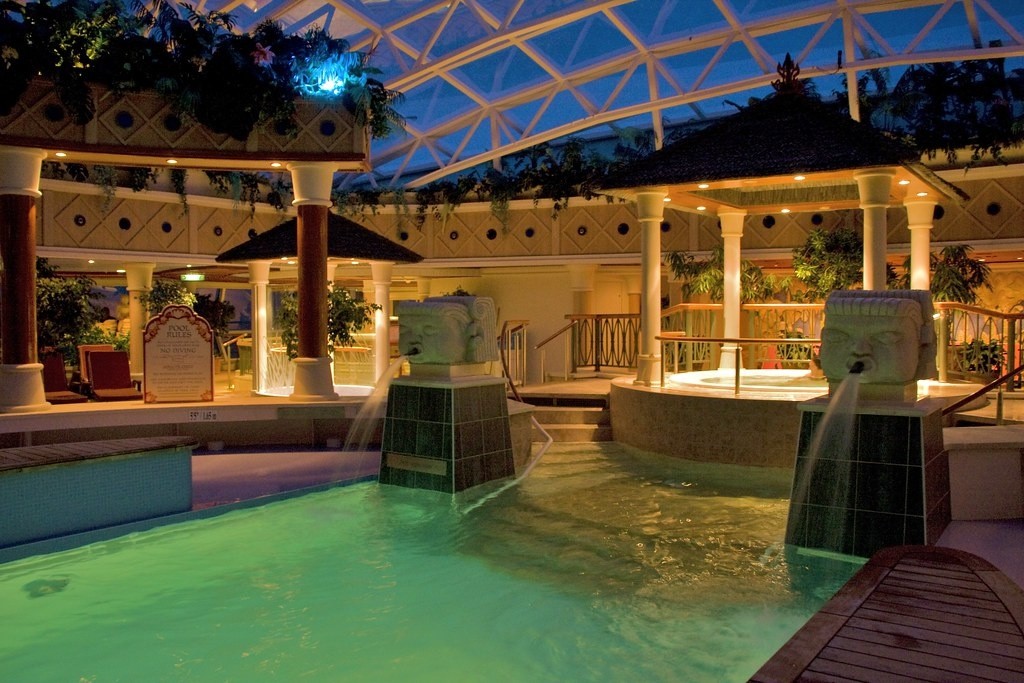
left=85, top=350, right=144, bottom=403
left=75, top=345, right=114, bottom=395
left=39, top=352, right=87, bottom=404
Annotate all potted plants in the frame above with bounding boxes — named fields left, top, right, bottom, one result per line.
left=679, top=333, right=710, bottom=371
left=776, top=333, right=817, bottom=369
left=193, top=292, right=236, bottom=375
left=953, top=338, right=1008, bottom=389
